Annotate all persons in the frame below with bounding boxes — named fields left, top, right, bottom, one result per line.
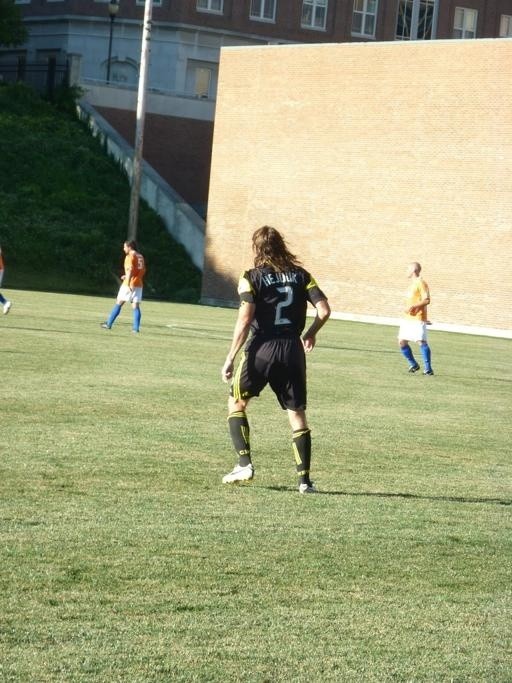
left=0, top=247, right=13, bottom=317
left=395, top=261, right=435, bottom=376
left=218, top=224, right=333, bottom=492
left=100, top=237, right=146, bottom=333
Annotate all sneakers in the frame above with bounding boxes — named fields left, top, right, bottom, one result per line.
left=100, top=323, right=111, bottom=329
left=222, top=463, right=254, bottom=483
left=2, top=301, right=12, bottom=316
left=408, top=364, right=434, bottom=376
left=299, top=482, right=318, bottom=494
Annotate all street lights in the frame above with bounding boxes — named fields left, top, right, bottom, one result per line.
left=107, top=0, right=119, bottom=82
